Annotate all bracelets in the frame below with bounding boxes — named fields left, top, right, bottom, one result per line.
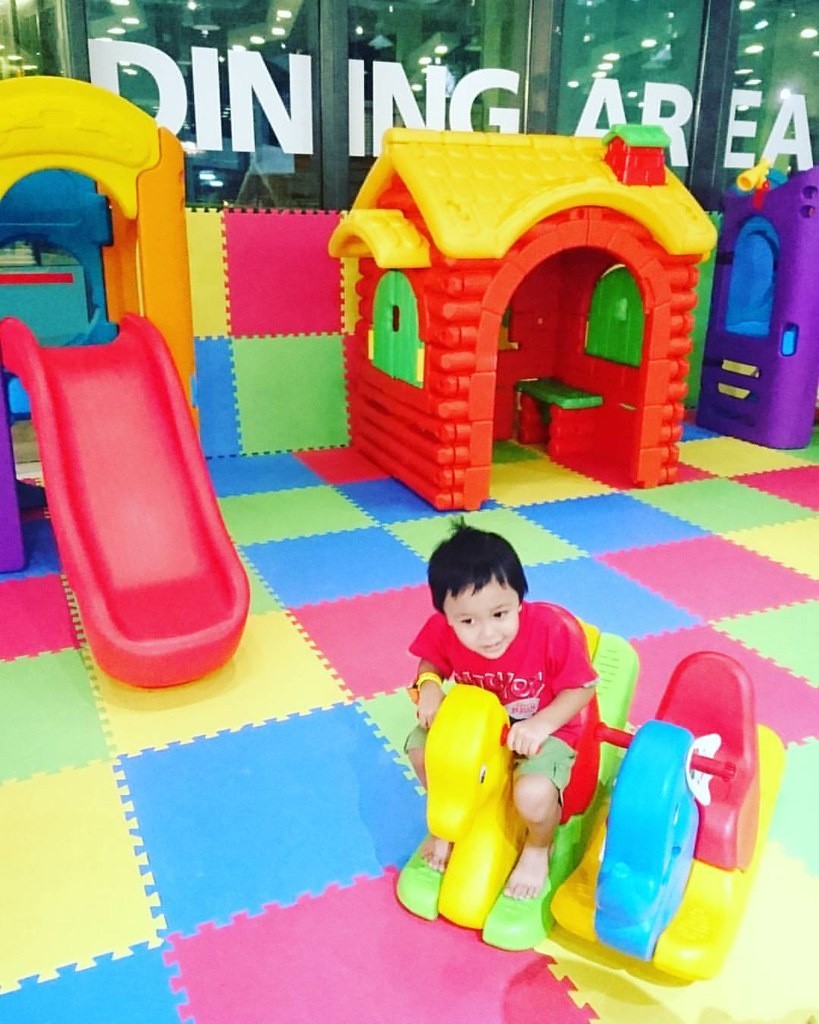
left=412, top=672, right=443, bottom=691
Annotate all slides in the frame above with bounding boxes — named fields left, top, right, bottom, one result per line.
left=0, top=309, right=251, bottom=690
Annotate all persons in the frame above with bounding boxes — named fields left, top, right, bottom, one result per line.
left=404, top=514, right=600, bottom=901
